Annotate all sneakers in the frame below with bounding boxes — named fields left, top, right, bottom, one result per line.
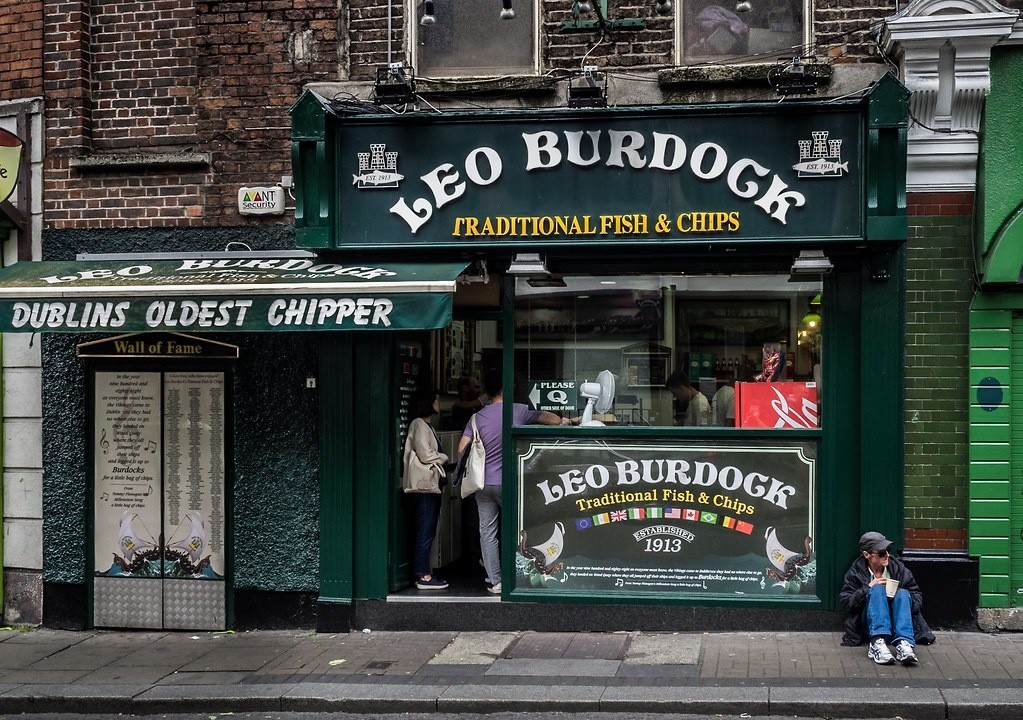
left=487, top=583, right=502, bottom=594
left=895, top=640, right=918, bottom=664
left=414, top=575, right=449, bottom=590
left=867, top=638, right=897, bottom=665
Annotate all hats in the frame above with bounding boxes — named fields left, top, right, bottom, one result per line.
left=858, top=532, right=895, bottom=553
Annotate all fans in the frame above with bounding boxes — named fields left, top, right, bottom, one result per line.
left=578, top=370, right=616, bottom=428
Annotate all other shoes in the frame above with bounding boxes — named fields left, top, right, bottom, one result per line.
left=485, top=576, right=490, bottom=582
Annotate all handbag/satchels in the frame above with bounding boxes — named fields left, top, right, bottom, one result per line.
left=460, top=413, right=486, bottom=499
left=404, top=420, right=446, bottom=494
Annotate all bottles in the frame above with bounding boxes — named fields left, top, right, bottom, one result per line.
left=714, top=357, right=741, bottom=380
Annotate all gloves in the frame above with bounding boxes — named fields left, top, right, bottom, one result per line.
left=477, top=394, right=489, bottom=407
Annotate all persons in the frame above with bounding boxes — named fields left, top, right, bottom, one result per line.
left=452, top=376, right=491, bottom=431
left=402, top=387, right=449, bottom=589
left=839, top=532, right=936, bottom=664
left=458, top=370, right=561, bottom=594
left=711, top=364, right=755, bottom=427
left=666, top=372, right=711, bottom=425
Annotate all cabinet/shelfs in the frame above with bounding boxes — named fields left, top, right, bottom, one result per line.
left=687, top=316, right=785, bottom=382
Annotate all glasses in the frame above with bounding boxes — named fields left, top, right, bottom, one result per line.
left=868, top=545, right=890, bottom=557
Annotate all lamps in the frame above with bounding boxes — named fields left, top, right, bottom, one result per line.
left=656, top=0, right=672, bottom=14
left=735, top=0, right=753, bottom=13
left=810, top=289, right=823, bottom=304
left=577, top=0, right=606, bottom=28
left=500, top=0, right=516, bottom=20
left=420, top=0, right=435, bottom=26
left=507, top=248, right=552, bottom=275
left=802, top=303, right=820, bottom=328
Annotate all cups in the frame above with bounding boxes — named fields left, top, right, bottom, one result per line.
left=886, top=579, right=900, bottom=598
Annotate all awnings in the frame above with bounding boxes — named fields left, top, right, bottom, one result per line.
left=0, top=261, right=489, bottom=333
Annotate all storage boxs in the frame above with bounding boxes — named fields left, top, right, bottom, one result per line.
left=706, top=25, right=736, bottom=54
left=747, top=29, right=798, bottom=53
